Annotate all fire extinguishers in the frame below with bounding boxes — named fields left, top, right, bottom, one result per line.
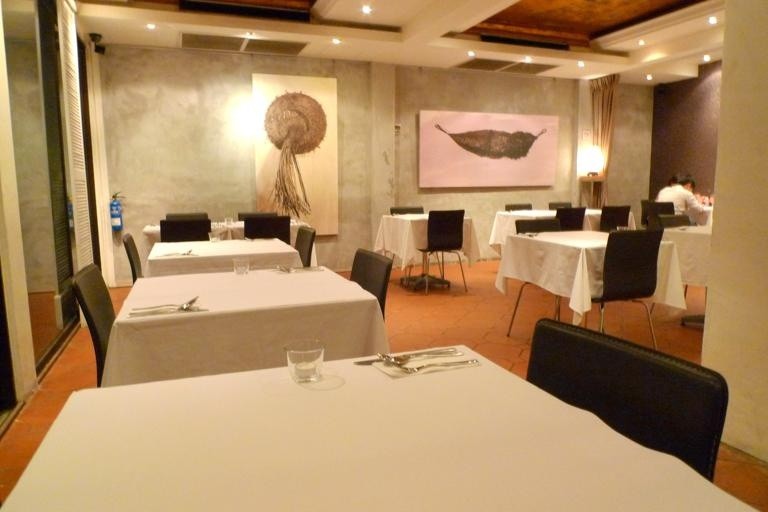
left=110, top=191, right=125, bottom=231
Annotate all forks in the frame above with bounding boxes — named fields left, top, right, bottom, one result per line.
left=393, top=357, right=478, bottom=374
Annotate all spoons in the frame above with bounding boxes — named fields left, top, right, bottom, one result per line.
left=373, top=347, right=465, bottom=367
left=127, top=295, right=199, bottom=309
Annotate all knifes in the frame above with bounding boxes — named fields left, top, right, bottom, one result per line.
left=128, top=308, right=208, bottom=315
left=354, top=348, right=457, bottom=366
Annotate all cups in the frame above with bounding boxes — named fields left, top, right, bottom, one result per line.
left=232, top=256, right=250, bottom=275
left=208, top=232, right=221, bottom=244
left=285, top=337, right=327, bottom=381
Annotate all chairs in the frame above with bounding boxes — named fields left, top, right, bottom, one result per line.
left=391, top=207, right=442, bottom=287
left=68, top=264, right=117, bottom=389
left=294, top=226, right=317, bottom=268
left=350, top=248, right=393, bottom=325
left=145, top=212, right=309, bottom=246
left=417, top=210, right=468, bottom=295
left=123, top=233, right=144, bottom=285
left=489, top=197, right=712, bottom=352
left=525, top=317, right=730, bottom=483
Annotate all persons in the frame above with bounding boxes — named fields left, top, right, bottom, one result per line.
left=669, top=175, right=680, bottom=186
left=654, top=174, right=713, bottom=214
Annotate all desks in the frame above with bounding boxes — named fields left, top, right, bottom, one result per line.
left=101, top=266, right=390, bottom=388
left=0, top=343, right=762, bottom=512
left=145, top=236, right=305, bottom=275
left=373, top=214, right=480, bottom=292
